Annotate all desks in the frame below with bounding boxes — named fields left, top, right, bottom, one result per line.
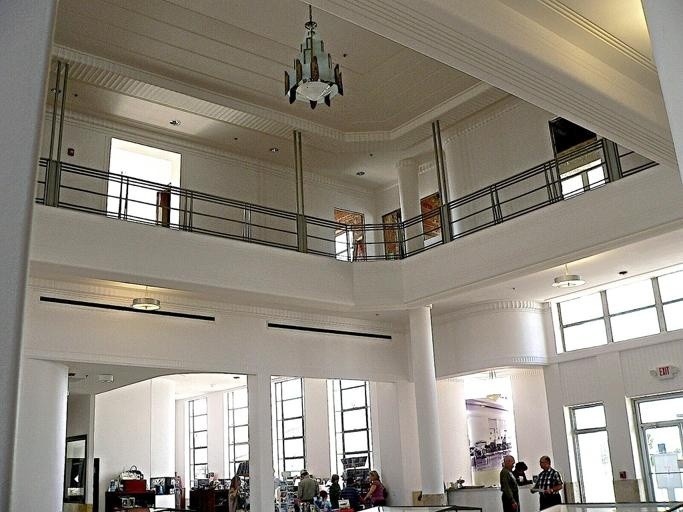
left=105, top=490, right=155, bottom=512
left=190, top=489, right=229, bottom=512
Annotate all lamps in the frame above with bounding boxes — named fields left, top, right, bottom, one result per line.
left=552, top=263, right=586, bottom=290
left=282, top=2, right=346, bottom=111
left=98, top=364, right=114, bottom=383
left=132, top=285, right=161, bottom=312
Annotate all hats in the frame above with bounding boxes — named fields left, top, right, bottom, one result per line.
left=300, top=469, right=307, bottom=475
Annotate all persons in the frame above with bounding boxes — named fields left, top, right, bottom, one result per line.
left=228, top=462, right=388, bottom=512
left=512, top=462, right=533, bottom=486
left=530, top=456, right=563, bottom=511
left=500, top=455, right=520, bottom=512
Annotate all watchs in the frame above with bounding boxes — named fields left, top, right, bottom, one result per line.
left=550, top=489, right=554, bottom=493
